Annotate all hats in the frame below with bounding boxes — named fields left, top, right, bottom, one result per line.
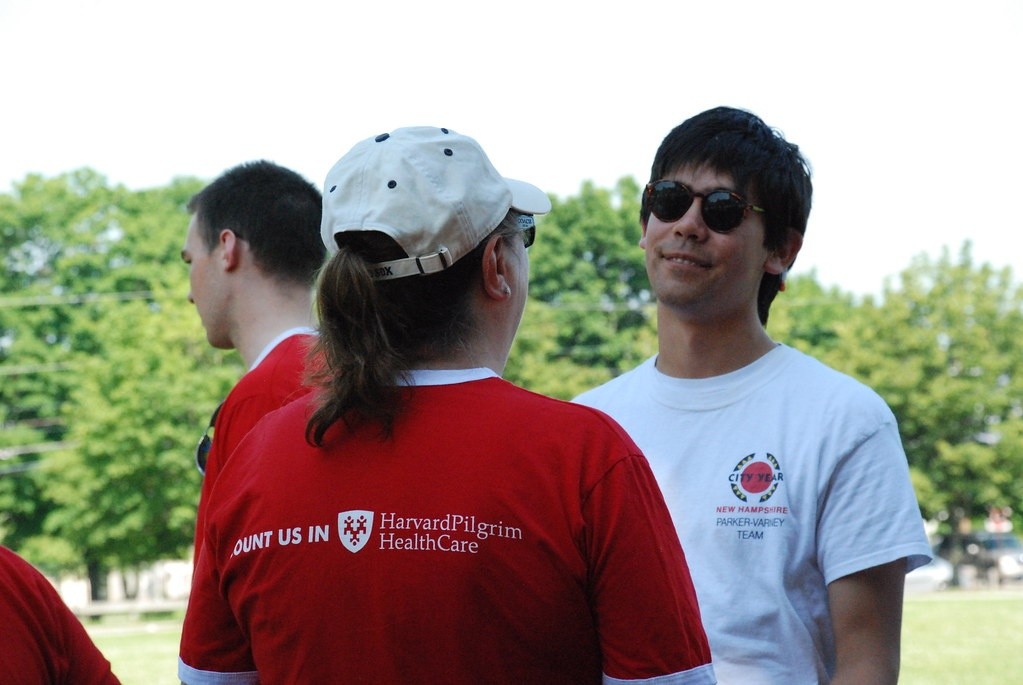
left=319, top=126, right=551, bottom=281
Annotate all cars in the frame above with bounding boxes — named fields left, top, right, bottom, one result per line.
left=934, top=532, right=1023, bottom=579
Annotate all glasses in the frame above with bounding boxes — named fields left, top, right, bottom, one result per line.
left=194, top=402, right=222, bottom=475
left=646, top=180, right=774, bottom=233
left=517, top=214, right=535, bottom=249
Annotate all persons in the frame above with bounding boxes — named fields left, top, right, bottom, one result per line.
left=0, top=546, right=122, bottom=685
left=571, top=107, right=934, bottom=685
left=177, top=126, right=717, bottom=685
left=180, top=160, right=332, bottom=477
